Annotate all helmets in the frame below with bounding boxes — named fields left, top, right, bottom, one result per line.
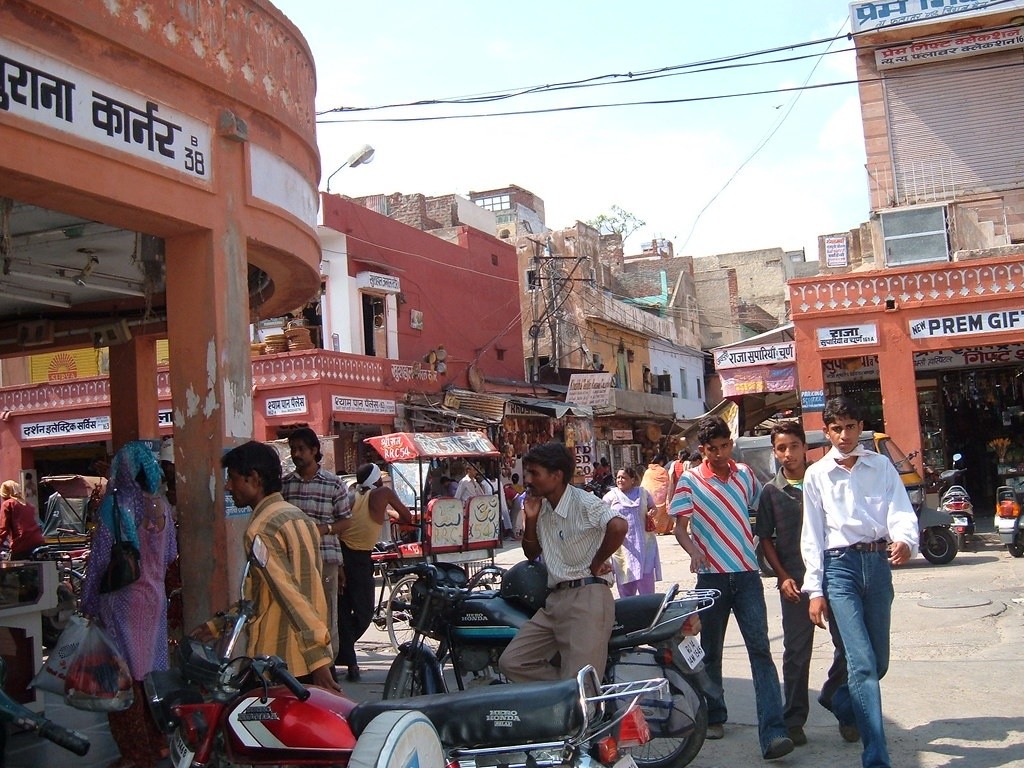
left=502, top=559, right=548, bottom=617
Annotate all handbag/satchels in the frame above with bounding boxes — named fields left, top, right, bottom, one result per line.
left=100, top=494, right=141, bottom=594
left=26, top=616, right=89, bottom=694
left=65, top=619, right=133, bottom=713
left=645, top=515, right=656, bottom=532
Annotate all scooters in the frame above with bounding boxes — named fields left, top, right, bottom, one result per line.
left=994, top=484, right=1024, bottom=558
left=923, top=452, right=976, bottom=551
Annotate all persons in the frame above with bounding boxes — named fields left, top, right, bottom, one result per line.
left=280, top=428, right=353, bottom=683
left=592, top=457, right=610, bottom=484
left=79, top=438, right=182, bottom=768
left=0, top=480, right=45, bottom=561
left=666, top=416, right=795, bottom=760
left=800, top=396, right=919, bottom=768
left=602, top=467, right=662, bottom=597
left=25, top=473, right=36, bottom=497
left=189, top=440, right=343, bottom=691
left=499, top=444, right=629, bottom=720
left=440, top=463, right=526, bottom=540
left=756, top=421, right=846, bottom=746
left=640, top=445, right=706, bottom=535
left=335, top=463, right=412, bottom=681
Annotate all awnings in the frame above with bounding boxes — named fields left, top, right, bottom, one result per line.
left=672, top=324, right=797, bottom=445
left=509, top=398, right=594, bottom=420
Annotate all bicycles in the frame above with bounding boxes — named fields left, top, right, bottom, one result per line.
left=0, top=536, right=89, bottom=651
left=367, top=538, right=445, bottom=660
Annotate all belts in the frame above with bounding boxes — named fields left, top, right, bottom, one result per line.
left=556, top=578, right=609, bottom=589
left=852, top=542, right=887, bottom=553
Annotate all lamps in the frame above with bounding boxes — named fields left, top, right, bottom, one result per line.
left=327, top=145, right=375, bottom=194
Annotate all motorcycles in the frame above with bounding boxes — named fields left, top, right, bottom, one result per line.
left=104, top=534, right=673, bottom=768
left=379, top=559, right=725, bottom=766
left=735, top=430, right=957, bottom=564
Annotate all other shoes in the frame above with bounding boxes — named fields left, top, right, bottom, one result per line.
left=789, top=727, right=808, bottom=745
left=764, top=736, right=794, bottom=758
left=349, top=664, right=360, bottom=683
left=839, top=720, right=861, bottom=742
left=818, top=695, right=832, bottom=714
left=703, top=723, right=724, bottom=739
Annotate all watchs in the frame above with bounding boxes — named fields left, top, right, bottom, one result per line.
left=326, top=524, right=332, bottom=535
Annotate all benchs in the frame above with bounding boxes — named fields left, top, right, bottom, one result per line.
left=396, top=494, right=499, bottom=556
